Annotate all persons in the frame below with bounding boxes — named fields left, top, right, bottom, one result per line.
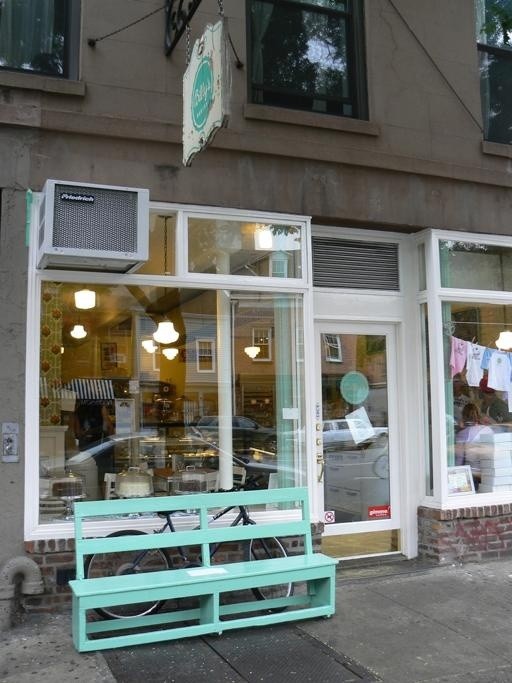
left=454, top=403, right=497, bottom=493
left=72, top=404, right=114, bottom=501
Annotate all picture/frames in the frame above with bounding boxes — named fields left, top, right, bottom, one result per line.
left=100, top=343, right=117, bottom=371
left=446, top=464, right=475, bottom=497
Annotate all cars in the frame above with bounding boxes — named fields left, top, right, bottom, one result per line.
left=40, top=433, right=278, bottom=488
left=282, top=419, right=389, bottom=444
left=193, top=416, right=277, bottom=453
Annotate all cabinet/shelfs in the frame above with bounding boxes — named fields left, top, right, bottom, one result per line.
left=326, top=450, right=389, bottom=520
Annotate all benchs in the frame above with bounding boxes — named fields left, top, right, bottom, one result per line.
left=67, top=485, right=339, bottom=654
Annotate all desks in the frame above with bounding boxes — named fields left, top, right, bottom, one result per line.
left=39, top=425, right=69, bottom=475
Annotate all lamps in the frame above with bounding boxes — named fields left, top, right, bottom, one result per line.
left=53, top=482, right=85, bottom=521
left=151, top=215, right=180, bottom=344
left=69, top=311, right=88, bottom=339
left=141, top=340, right=158, bottom=354
left=162, top=347, right=178, bottom=361
left=74, top=283, right=96, bottom=310
left=494, top=247, right=512, bottom=351
left=244, top=346, right=261, bottom=359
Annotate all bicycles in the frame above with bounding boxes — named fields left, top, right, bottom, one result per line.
left=372, top=432, right=390, bottom=480
left=82, top=472, right=305, bottom=620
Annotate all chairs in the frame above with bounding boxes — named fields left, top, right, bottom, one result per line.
left=105, top=475, right=123, bottom=500
left=233, top=466, right=247, bottom=484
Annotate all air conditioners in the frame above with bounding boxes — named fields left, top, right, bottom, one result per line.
left=37, top=179, right=149, bottom=274
